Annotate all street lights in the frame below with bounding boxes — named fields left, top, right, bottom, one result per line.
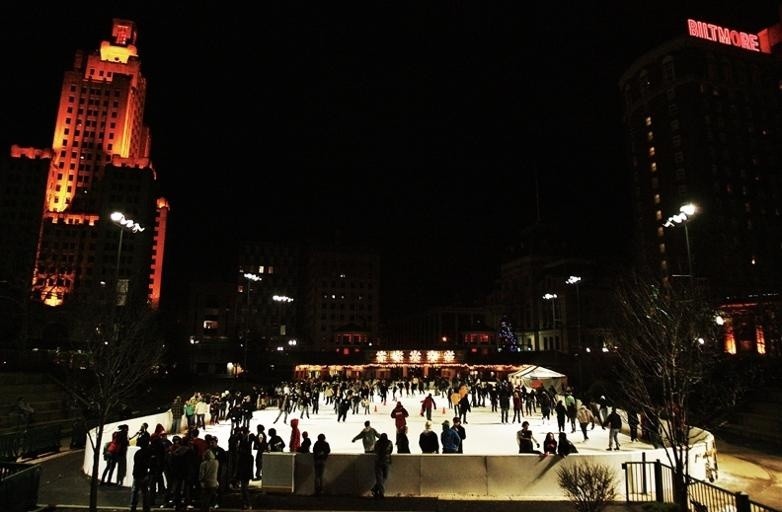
left=271, top=294, right=296, bottom=367
left=542, top=292, right=559, bottom=349
left=108, top=206, right=145, bottom=353
left=241, top=268, right=266, bottom=375
left=564, top=274, right=585, bottom=354
left=662, top=200, right=704, bottom=372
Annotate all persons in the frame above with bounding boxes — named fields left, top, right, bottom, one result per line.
left=101, top=374, right=665, bottom=511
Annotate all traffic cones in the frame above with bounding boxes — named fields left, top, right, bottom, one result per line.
left=443, top=408, right=445, bottom=414
left=374, top=406, right=377, bottom=412
